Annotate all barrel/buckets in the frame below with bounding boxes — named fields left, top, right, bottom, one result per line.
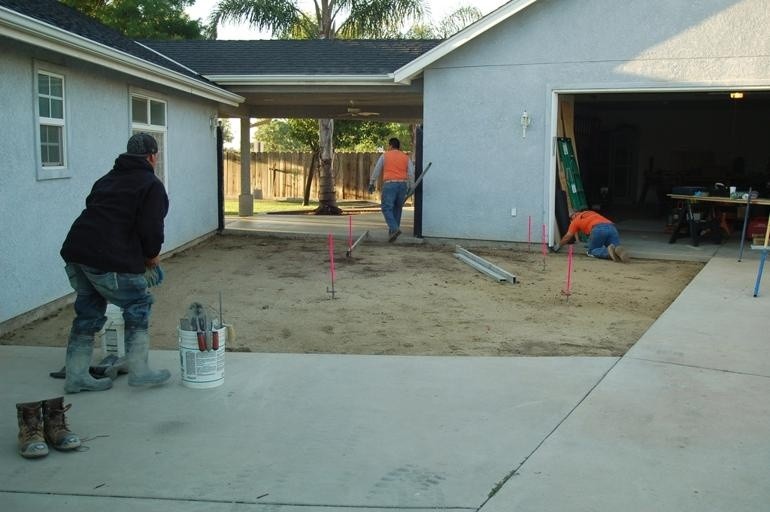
left=98, top=305, right=124, bottom=360
left=176, top=326, right=227, bottom=390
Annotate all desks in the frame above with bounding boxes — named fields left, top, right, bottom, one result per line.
left=666, top=193, right=769, bottom=246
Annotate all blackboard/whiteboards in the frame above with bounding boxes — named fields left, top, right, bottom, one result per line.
left=557, top=137, right=591, bottom=243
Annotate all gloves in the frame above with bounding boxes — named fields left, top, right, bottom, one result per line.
left=143, top=265, right=165, bottom=289
left=552, top=243, right=561, bottom=253
left=367, top=184, right=376, bottom=194
left=407, top=187, right=415, bottom=196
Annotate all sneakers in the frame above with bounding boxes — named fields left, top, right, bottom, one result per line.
left=388, top=229, right=403, bottom=243
left=607, top=243, right=631, bottom=264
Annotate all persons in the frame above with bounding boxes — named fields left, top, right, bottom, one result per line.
left=368, top=138, right=413, bottom=242
left=552, top=211, right=628, bottom=261
left=60, top=133, right=169, bottom=392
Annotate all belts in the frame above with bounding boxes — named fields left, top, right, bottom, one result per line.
left=383, top=179, right=407, bottom=184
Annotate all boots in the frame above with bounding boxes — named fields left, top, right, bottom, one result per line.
left=15, top=395, right=83, bottom=459
left=123, top=326, right=173, bottom=388
left=63, top=330, right=116, bottom=394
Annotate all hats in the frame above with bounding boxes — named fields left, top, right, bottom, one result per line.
left=567, top=207, right=579, bottom=218
left=120, top=131, right=159, bottom=159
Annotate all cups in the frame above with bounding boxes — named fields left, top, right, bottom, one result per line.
left=729, top=187, right=736, bottom=199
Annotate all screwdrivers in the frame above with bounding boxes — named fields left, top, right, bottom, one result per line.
left=195, top=315, right=206, bottom=351
